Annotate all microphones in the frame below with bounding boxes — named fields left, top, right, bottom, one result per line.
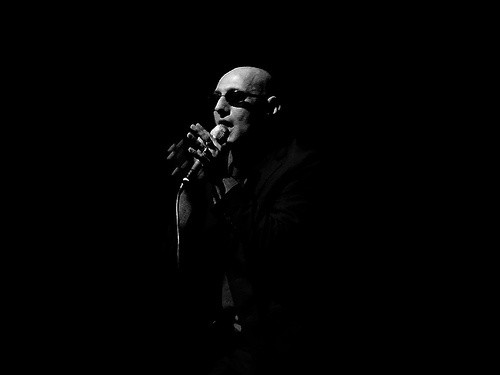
left=182, top=124, right=231, bottom=188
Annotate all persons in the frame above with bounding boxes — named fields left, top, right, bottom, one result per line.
left=166, top=66, right=309, bottom=375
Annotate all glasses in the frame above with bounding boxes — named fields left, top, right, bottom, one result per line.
left=211, top=89, right=272, bottom=107
